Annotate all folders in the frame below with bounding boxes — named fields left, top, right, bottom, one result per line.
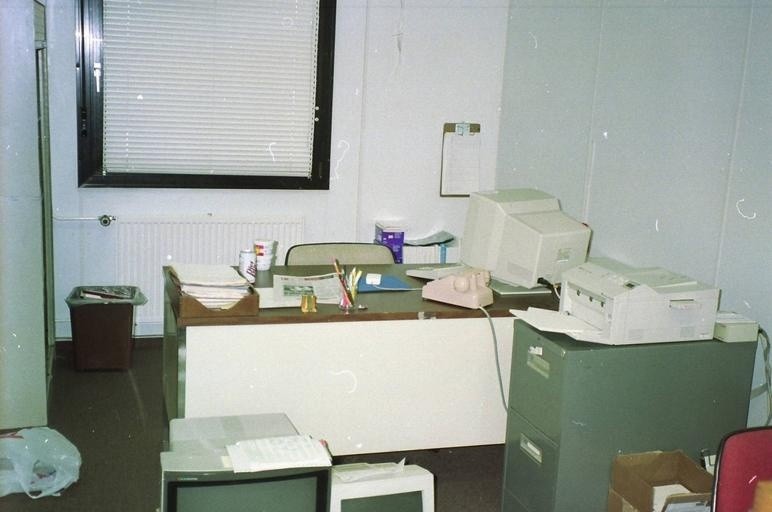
left=357, top=274, right=410, bottom=292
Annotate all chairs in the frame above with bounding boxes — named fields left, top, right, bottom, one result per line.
left=284, top=243, right=399, bottom=266
left=711, top=427, right=772, bottom=512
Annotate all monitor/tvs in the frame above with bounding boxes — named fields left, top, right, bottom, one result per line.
left=330, top=462, right=435, bottom=512
left=461, top=188, right=593, bottom=289
left=157, top=411, right=332, bottom=512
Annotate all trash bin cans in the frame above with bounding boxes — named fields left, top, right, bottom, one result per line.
left=66, top=286, right=138, bottom=369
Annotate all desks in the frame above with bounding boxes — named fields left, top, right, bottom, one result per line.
left=160, top=264, right=561, bottom=456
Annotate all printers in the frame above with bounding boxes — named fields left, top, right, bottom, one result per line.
left=557, top=261, right=722, bottom=345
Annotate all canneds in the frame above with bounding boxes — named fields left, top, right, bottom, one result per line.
left=238, top=250, right=256, bottom=284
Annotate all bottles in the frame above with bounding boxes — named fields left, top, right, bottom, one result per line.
left=301, top=285, right=319, bottom=314
left=338, top=282, right=358, bottom=312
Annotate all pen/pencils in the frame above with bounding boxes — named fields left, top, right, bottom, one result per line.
left=334, top=257, right=363, bottom=312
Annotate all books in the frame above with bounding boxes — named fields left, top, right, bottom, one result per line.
left=170, top=264, right=253, bottom=310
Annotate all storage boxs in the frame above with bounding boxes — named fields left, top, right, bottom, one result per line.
left=607, top=449, right=715, bottom=512
left=374, top=219, right=404, bottom=263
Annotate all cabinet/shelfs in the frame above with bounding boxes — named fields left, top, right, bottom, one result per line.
left=500, top=319, right=759, bottom=512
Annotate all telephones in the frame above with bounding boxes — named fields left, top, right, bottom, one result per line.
left=422, top=269, right=493, bottom=309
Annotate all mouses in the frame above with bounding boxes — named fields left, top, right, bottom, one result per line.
left=365, top=272, right=383, bottom=285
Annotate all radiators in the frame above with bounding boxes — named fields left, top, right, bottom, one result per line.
left=113, top=217, right=306, bottom=338
